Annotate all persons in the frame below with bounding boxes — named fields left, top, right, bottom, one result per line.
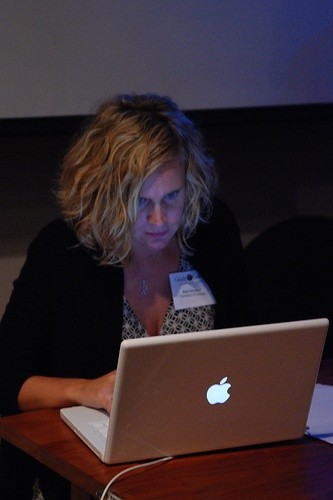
left=0, top=92, right=264, bottom=500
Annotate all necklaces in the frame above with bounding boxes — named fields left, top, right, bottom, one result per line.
left=124, top=259, right=160, bottom=295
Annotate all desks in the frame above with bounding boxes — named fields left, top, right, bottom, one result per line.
left=0, top=354, right=333, bottom=500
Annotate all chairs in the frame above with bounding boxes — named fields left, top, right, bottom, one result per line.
left=212, top=196, right=333, bottom=359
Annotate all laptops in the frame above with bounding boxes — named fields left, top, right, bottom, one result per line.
left=60, top=318, right=329, bottom=465
